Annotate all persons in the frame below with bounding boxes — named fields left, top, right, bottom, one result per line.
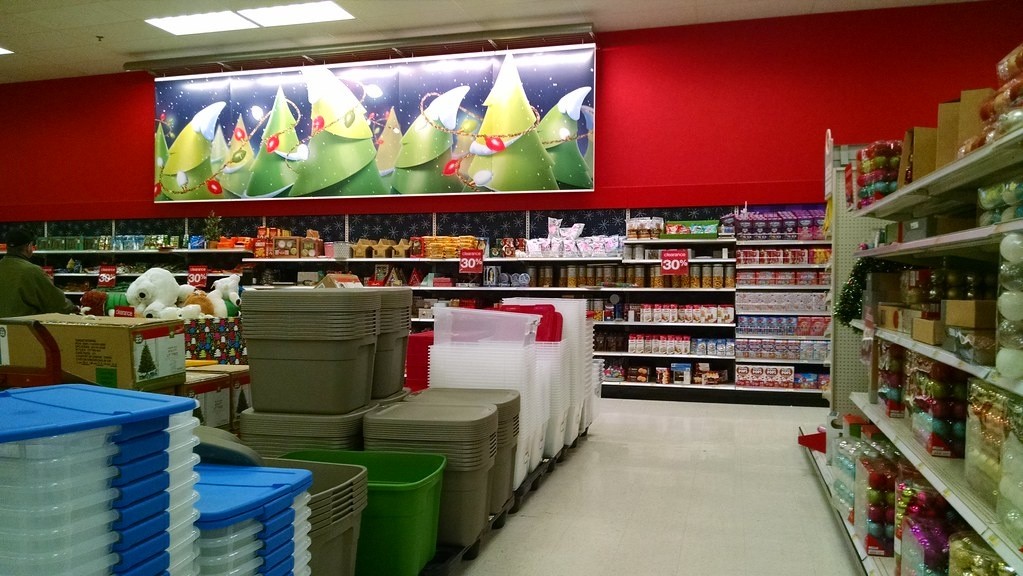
left=0, top=223, right=80, bottom=318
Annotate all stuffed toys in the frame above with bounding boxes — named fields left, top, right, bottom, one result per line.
left=83, top=265, right=242, bottom=322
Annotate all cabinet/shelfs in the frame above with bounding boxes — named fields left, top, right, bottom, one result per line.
left=799, top=121, right=1023, bottom=575
left=25, top=247, right=830, bottom=401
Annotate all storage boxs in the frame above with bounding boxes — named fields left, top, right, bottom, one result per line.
left=895, top=85, right=998, bottom=195
left=733, top=210, right=831, bottom=389
left=868, top=301, right=997, bottom=369
left=625, top=305, right=734, bottom=358
left=489, top=238, right=526, bottom=258
left=254, top=226, right=351, bottom=258
left=0, top=281, right=603, bottom=576
left=627, top=363, right=728, bottom=386
